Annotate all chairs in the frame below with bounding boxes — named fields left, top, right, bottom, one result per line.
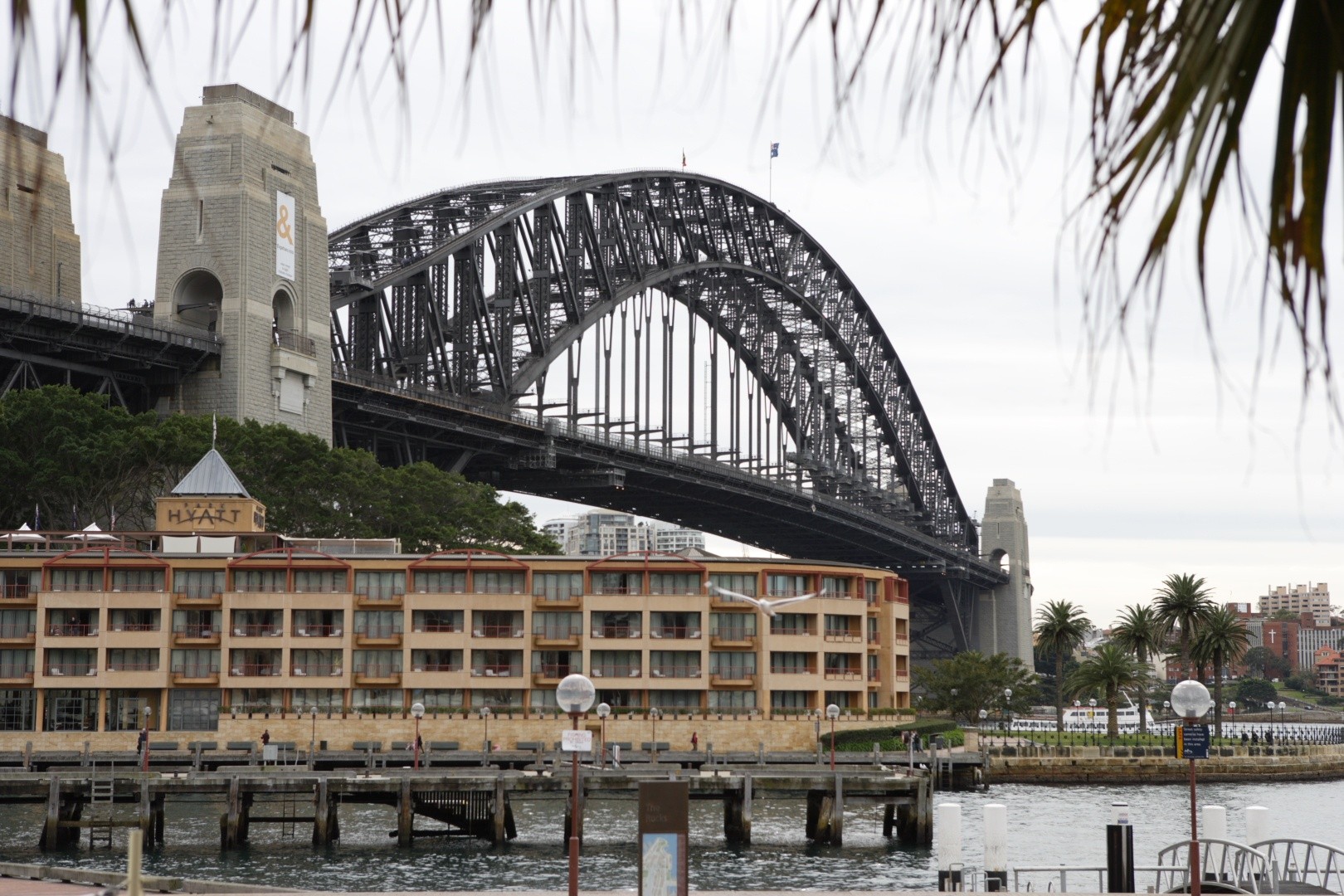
left=591, top=628, right=702, bottom=678
left=414, top=627, right=524, bottom=678
left=231, top=628, right=343, bottom=677
left=51, top=627, right=122, bottom=677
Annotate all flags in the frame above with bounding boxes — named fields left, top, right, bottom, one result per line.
left=212, top=409, right=217, bottom=439
left=72, top=504, right=78, bottom=531
left=683, top=149, right=687, bottom=166
left=111, top=505, right=115, bottom=531
left=35, top=504, right=40, bottom=531
left=771, top=143, right=779, bottom=158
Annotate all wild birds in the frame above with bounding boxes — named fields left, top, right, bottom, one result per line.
left=920, top=763, right=927, bottom=770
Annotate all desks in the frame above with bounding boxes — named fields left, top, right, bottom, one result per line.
left=262, top=632, right=272, bottom=637
left=261, top=670, right=270, bottom=675
left=74, top=671, right=84, bottom=677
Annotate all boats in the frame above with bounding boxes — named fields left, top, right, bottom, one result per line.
left=1011, top=706, right=1175, bottom=737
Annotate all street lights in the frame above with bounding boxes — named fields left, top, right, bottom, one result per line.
left=310, top=706, right=318, bottom=741
left=1171, top=680, right=1210, bottom=896
left=556, top=672, right=595, bottom=896
left=1268, top=701, right=1274, bottom=738
left=141, top=707, right=152, bottom=772
left=1208, top=700, right=1216, bottom=737
left=1279, top=702, right=1286, bottom=745
left=1163, top=701, right=1170, bottom=736
left=1229, top=702, right=1236, bottom=739
left=1005, top=689, right=1012, bottom=737
left=597, top=703, right=611, bottom=771
left=814, top=709, right=822, bottom=743
left=951, top=689, right=958, bottom=721
left=978, top=709, right=987, bottom=757
left=1088, top=699, right=1097, bottom=735
left=826, top=704, right=840, bottom=770
left=411, top=702, right=426, bottom=770
left=1074, top=700, right=1080, bottom=733
left=917, top=696, right=922, bottom=718
left=650, top=707, right=658, bottom=764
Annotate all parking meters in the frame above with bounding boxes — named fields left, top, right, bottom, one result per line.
left=482, top=707, right=491, bottom=741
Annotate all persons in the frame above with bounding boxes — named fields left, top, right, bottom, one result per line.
left=137, top=728, right=147, bottom=756
left=297, top=706, right=304, bottom=719
left=901, top=729, right=924, bottom=752
left=418, top=733, right=424, bottom=753
left=1241, top=731, right=1273, bottom=746
left=261, top=730, right=270, bottom=745
left=691, top=732, right=697, bottom=750
